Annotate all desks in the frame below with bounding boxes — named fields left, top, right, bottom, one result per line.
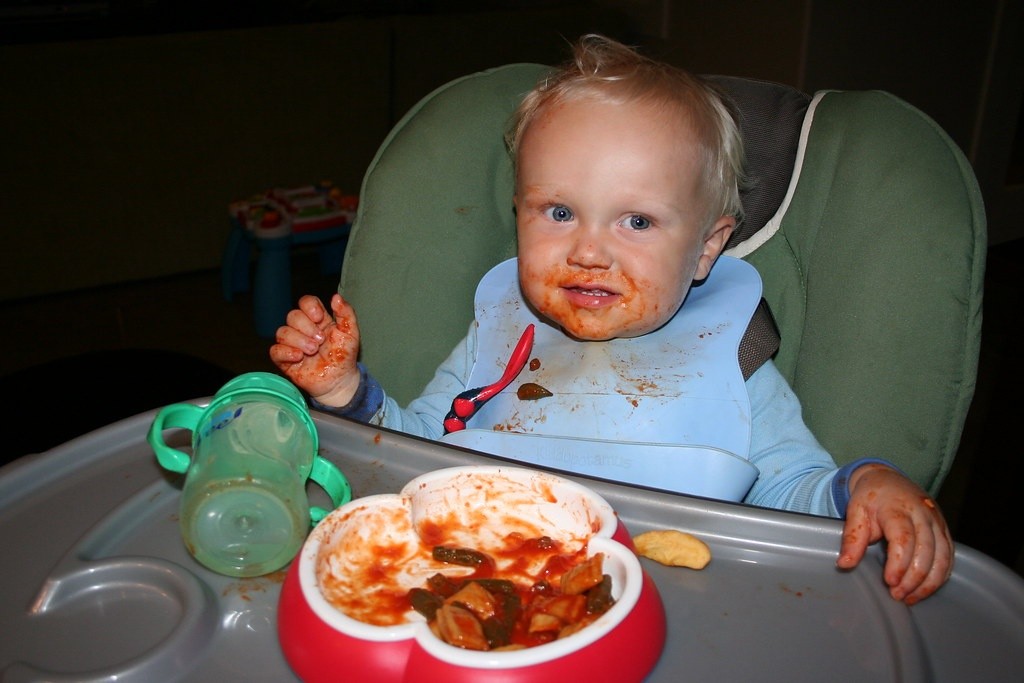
left=0, top=389, right=1024, bottom=682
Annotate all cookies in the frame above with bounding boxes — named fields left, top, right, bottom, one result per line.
left=633, top=531, right=711, bottom=571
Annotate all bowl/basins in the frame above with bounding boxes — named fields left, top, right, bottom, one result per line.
left=278, top=464, right=668, bottom=683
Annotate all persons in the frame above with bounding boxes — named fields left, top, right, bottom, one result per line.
left=270, top=32, right=954, bottom=606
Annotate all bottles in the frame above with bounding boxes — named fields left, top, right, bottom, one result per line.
left=147, top=372, right=352, bottom=578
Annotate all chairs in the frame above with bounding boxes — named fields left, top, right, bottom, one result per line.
left=337, top=64, right=988, bottom=502
